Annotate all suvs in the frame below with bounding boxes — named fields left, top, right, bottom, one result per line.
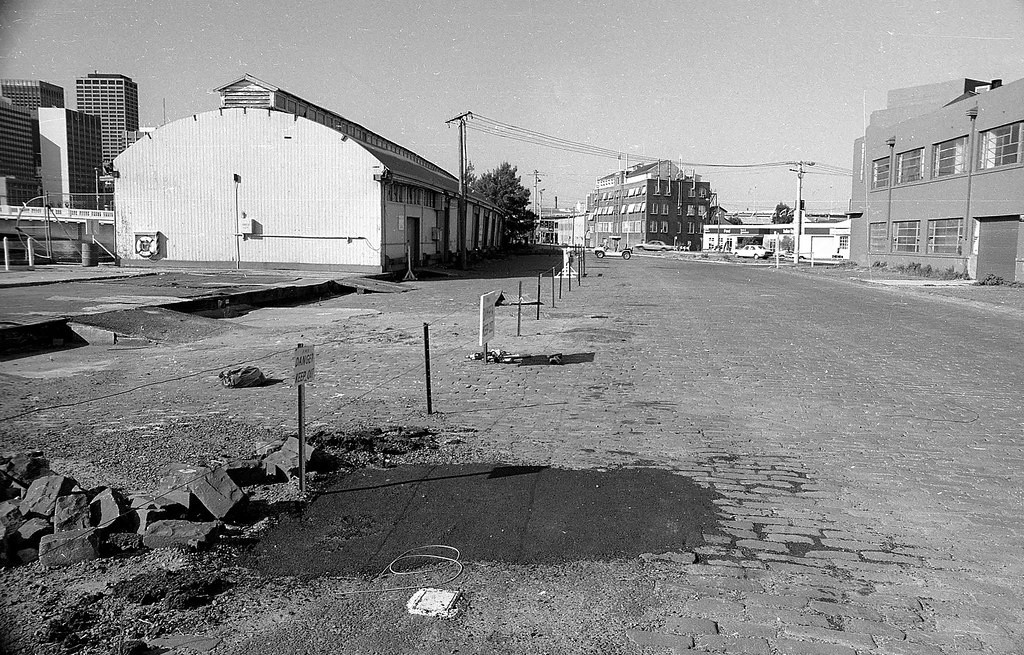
left=594, top=236, right=633, bottom=260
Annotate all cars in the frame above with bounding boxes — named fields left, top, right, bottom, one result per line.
left=733, top=243, right=772, bottom=260
left=777, top=249, right=807, bottom=260
left=634, top=239, right=676, bottom=252
